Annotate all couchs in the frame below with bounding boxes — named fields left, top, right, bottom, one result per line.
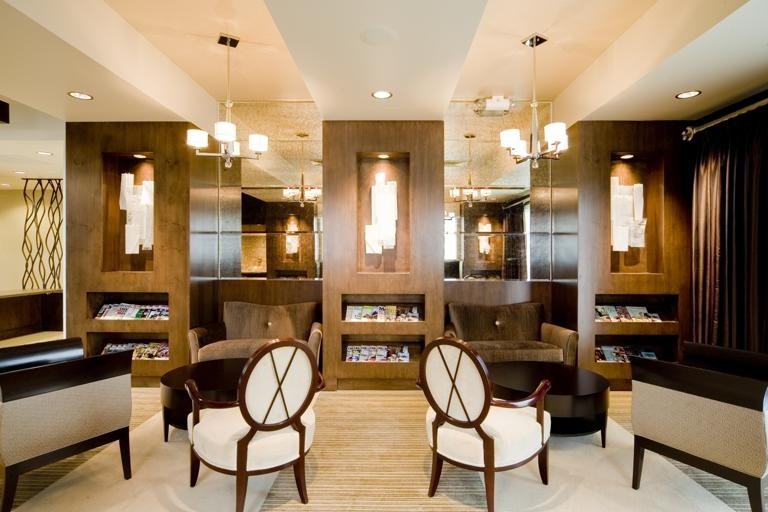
left=449, top=300, right=578, bottom=366
left=0, top=338, right=135, bottom=511
left=419, top=329, right=552, bottom=512
left=187, top=299, right=323, bottom=366
left=631, top=340, right=768, bottom=511
left=184, top=338, right=326, bottom=512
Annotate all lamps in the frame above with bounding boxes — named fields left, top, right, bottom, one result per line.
left=281, top=133, right=322, bottom=207
left=500, top=33, right=569, bottom=169
left=450, top=135, right=491, bottom=208
left=187, top=34, right=268, bottom=167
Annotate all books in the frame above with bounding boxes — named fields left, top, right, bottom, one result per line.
left=344, top=304, right=424, bottom=364
left=595, top=306, right=662, bottom=363
left=94, top=302, right=170, bottom=360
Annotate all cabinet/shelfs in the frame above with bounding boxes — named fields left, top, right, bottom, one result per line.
left=84, top=289, right=171, bottom=387
left=336, top=292, right=425, bottom=389
left=595, top=293, right=682, bottom=390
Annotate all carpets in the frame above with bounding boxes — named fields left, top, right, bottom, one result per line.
left=475, top=417, right=735, bottom=512
left=13, top=412, right=281, bottom=512
